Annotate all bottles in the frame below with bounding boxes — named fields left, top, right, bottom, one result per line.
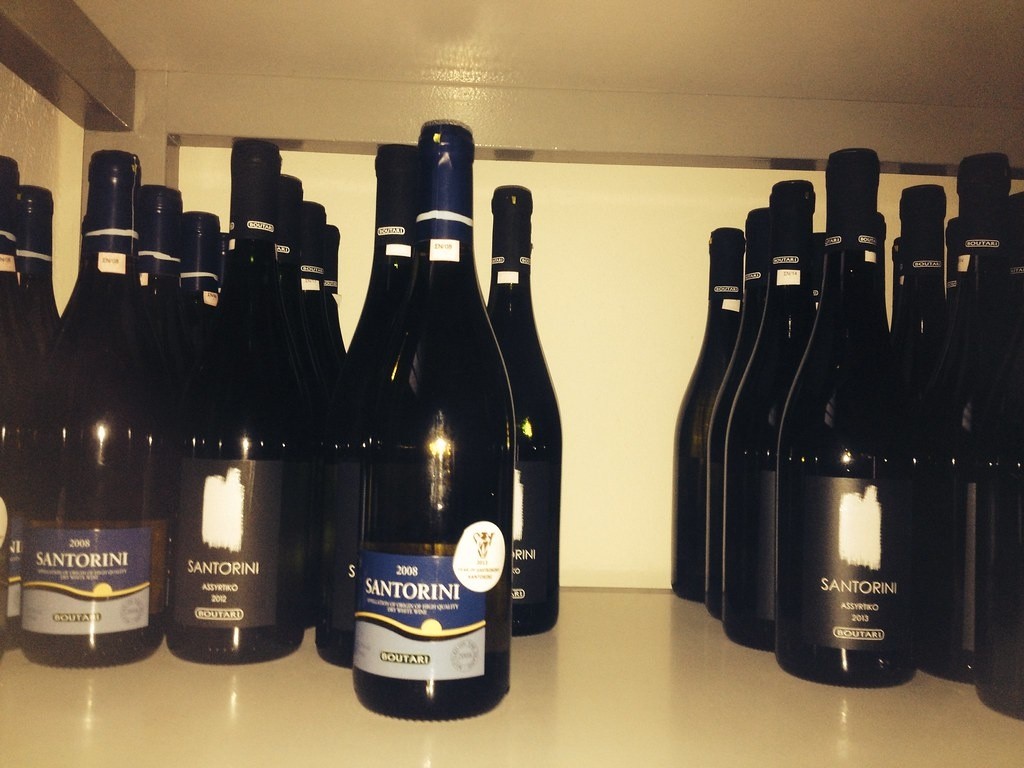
left=773, top=150, right=919, bottom=689
left=314, top=143, right=427, bottom=670
left=705, top=208, right=771, bottom=623
left=670, top=228, right=745, bottom=602
left=0, top=136, right=346, bottom=669
left=355, top=121, right=516, bottom=719
left=487, top=186, right=563, bottom=636
left=808, top=148, right=1024, bottom=718
left=720, top=179, right=815, bottom=655
